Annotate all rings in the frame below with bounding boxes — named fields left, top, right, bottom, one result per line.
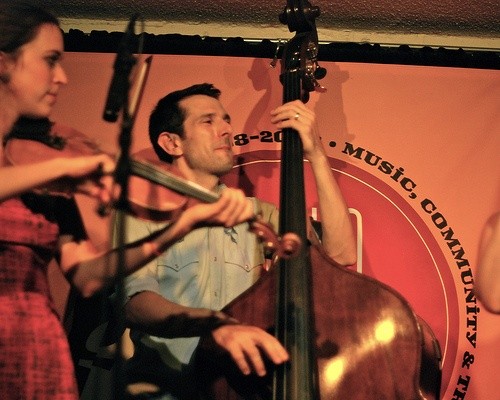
left=294, top=113, right=300, bottom=120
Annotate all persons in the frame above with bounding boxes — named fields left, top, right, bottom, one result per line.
left=0, top=0, right=257, bottom=400
left=103, top=79, right=359, bottom=378
left=472, top=209, right=500, bottom=315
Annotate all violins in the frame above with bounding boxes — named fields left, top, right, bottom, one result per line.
left=5, top=117, right=302, bottom=258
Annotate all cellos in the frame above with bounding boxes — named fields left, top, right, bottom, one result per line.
left=195, top=0, right=441, bottom=400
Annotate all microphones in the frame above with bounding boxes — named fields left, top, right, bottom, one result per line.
left=103, top=40, right=131, bottom=121
